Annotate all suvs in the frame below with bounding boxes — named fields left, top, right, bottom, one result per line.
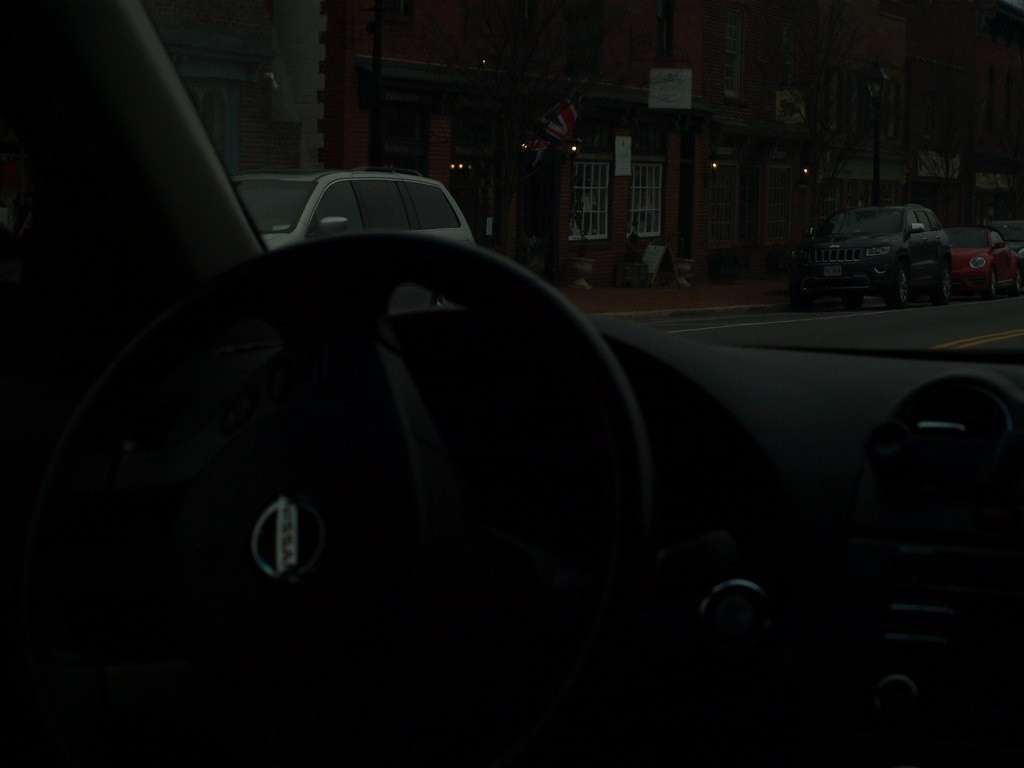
left=789, top=204, right=953, bottom=310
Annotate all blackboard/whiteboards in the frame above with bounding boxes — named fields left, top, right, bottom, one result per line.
left=642, top=241, right=677, bottom=274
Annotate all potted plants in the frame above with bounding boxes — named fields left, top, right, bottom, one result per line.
left=673, top=230, right=694, bottom=285
left=559, top=187, right=596, bottom=290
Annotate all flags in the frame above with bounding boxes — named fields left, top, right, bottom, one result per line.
left=513, top=87, right=581, bottom=168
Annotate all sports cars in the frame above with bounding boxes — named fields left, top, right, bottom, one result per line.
left=944, top=220, right=1024, bottom=299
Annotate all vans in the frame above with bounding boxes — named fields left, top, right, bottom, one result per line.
left=232, top=170, right=476, bottom=311
